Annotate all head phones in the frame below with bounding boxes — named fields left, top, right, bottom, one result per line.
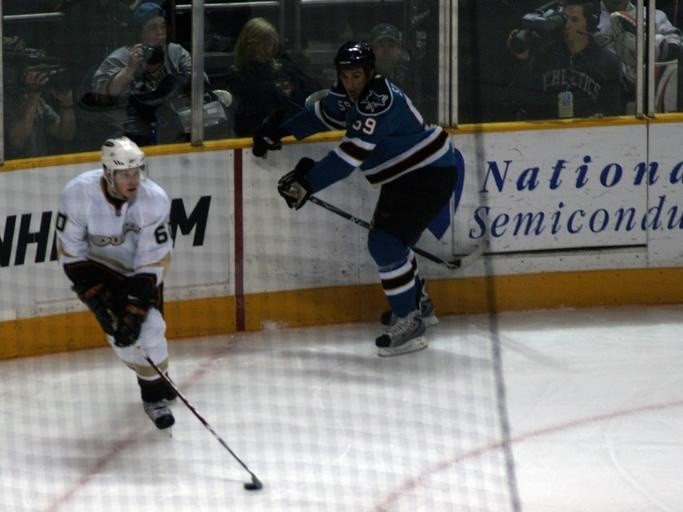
left=587, top=13, right=600, bottom=32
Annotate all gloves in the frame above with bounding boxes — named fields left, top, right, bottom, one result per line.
left=278, top=157, right=315, bottom=209
left=115, top=299, right=149, bottom=347
left=252, top=113, right=282, bottom=157
left=81, top=284, right=120, bottom=335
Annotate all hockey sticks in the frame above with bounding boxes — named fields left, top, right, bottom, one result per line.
left=308, top=196, right=490, bottom=271
left=106, top=308, right=263, bottom=489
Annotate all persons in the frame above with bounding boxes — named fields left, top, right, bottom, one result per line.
left=53, top=135, right=177, bottom=430
left=1, top=1, right=682, bottom=162
left=250, top=38, right=459, bottom=349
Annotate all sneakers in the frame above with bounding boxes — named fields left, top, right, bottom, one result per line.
left=142, top=384, right=177, bottom=429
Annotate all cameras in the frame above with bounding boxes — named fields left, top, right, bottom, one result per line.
left=140, top=45, right=165, bottom=65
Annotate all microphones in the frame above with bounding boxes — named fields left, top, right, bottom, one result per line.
left=575, top=31, right=595, bottom=38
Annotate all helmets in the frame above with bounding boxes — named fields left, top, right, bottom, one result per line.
left=334, top=39, right=374, bottom=70
left=101, top=136, right=146, bottom=193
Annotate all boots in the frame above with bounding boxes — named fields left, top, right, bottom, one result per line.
left=374, top=294, right=425, bottom=347
left=381, top=274, right=434, bottom=325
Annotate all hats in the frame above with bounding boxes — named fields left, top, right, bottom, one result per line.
left=133, top=2, right=167, bottom=23
left=363, top=21, right=402, bottom=50
